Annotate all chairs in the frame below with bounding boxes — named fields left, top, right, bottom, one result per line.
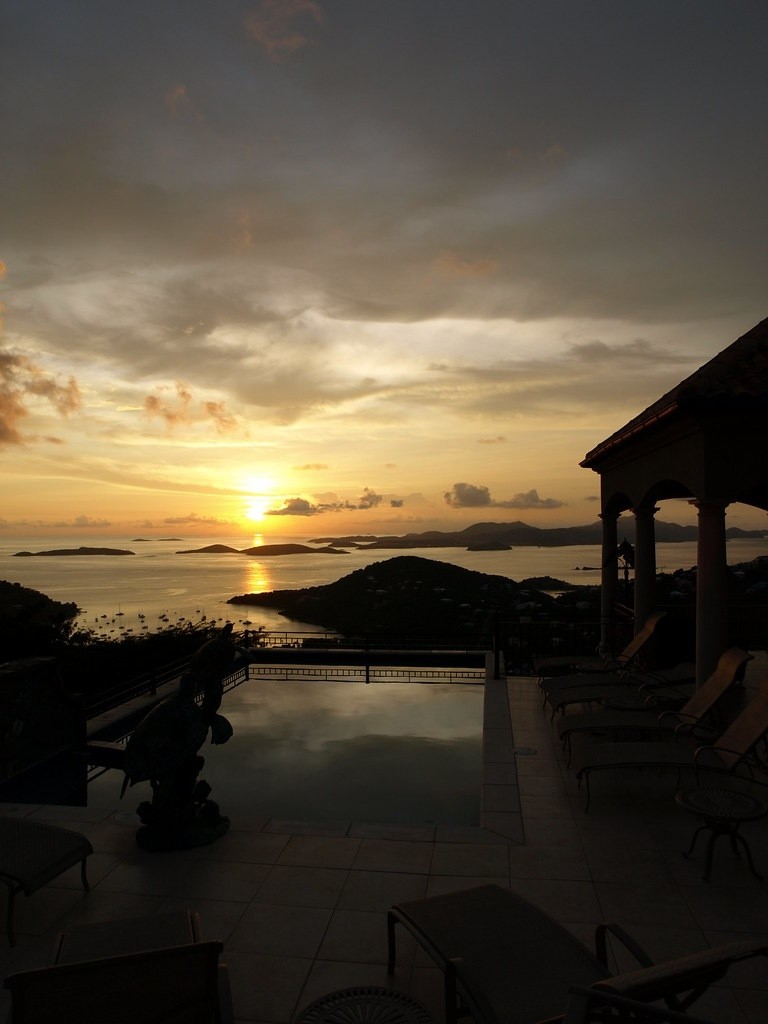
left=0, top=816, right=93, bottom=948
left=534, top=608, right=768, bottom=819
left=384, top=882, right=768, bottom=1024
left=6, top=908, right=236, bottom=1024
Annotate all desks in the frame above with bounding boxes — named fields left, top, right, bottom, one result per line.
left=601, top=694, right=659, bottom=743
left=294, top=985, right=436, bottom=1024
left=674, top=784, right=768, bottom=881
